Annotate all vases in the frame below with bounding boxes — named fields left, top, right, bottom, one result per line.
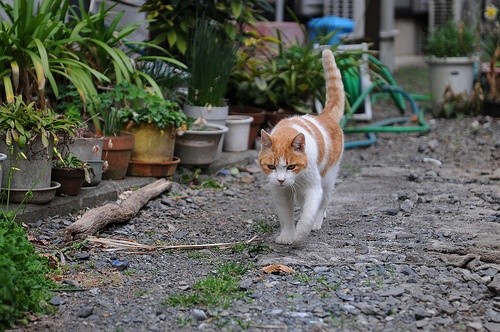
left=479, top=60, right=500, bottom=106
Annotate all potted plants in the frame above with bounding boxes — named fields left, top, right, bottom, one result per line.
left=422, top=17, right=481, bottom=119
left=0, top=0, right=392, bottom=189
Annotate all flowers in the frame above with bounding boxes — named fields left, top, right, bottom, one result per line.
left=476, top=2, right=499, bottom=64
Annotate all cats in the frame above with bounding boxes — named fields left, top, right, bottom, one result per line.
left=258, top=48, right=345, bottom=248
